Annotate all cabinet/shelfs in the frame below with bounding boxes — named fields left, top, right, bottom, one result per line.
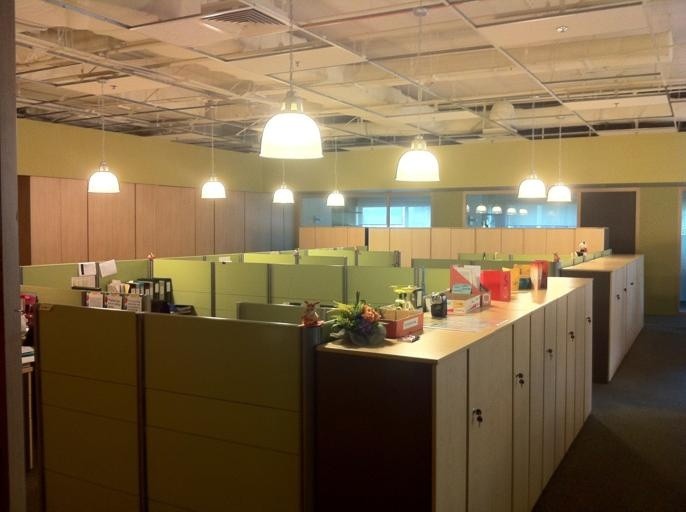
left=627, top=256, right=645, bottom=350
left=545, top=299, right=557, bottom=488
left=614, top=255, right=627, bottom=375
left=565, top=288, right=575, bottom=454
left=585, top=278, right=593, bottom=422
left=466, top=321, right=513, bottom=512
left=513, top=314, right=531, bottom=512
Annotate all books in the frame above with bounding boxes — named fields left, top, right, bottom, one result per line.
left=107, top=278, right=196, bottom=316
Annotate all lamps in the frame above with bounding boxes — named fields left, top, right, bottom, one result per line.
left=87, top=79, right=120, bottom=194
left=271, top=156, right=296, bottom=205
left=548, top=111, right=572, bottom=202
left=326, top=136, right=345, bottom=207
left=258, top=0, right=324, bottom=160
left=516, top=94, right=546, bottom=199
left=394, top=81, right=440, bottom=182
left=200, top=100, right=227, bottom=199
